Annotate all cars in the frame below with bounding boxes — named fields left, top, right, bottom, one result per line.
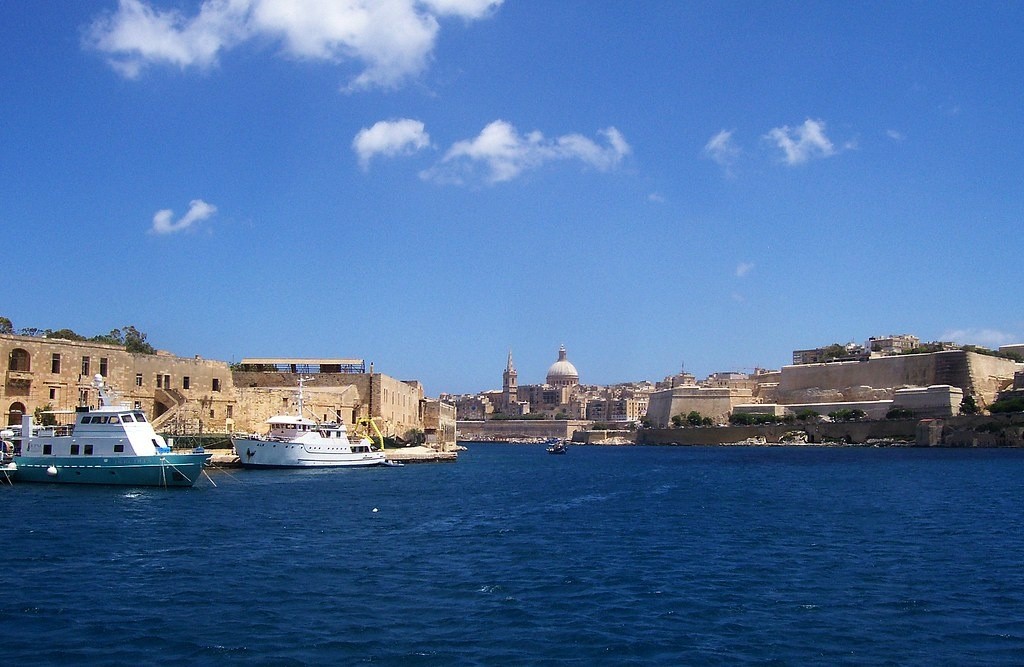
left=6, top=422, right=75, bottom=436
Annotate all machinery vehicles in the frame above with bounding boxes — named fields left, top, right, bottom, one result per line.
left=349, top=415, right=385, bottom=452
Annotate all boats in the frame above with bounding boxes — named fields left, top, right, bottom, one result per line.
left=231, top=376, right=406, bottom=467
left=11, top=375, right=213, bottom=488
left=546, top=442, right=567, bottom=455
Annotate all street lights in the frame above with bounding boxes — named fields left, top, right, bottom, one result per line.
left=297, top=372, right=316, bottom=417
